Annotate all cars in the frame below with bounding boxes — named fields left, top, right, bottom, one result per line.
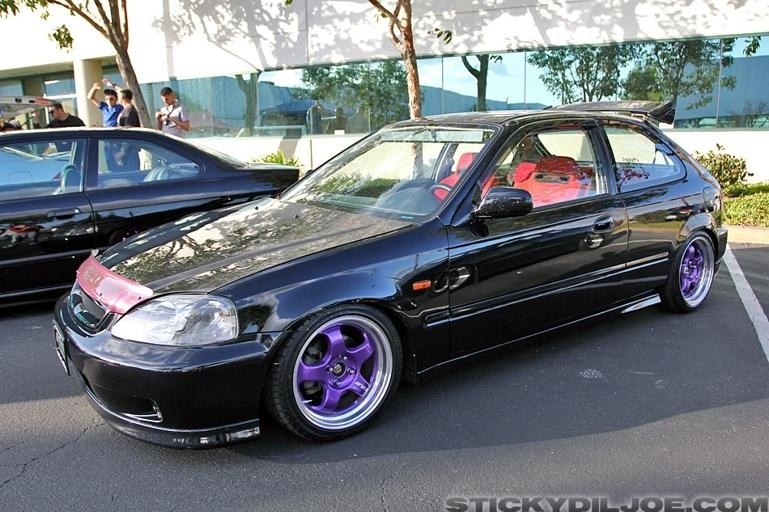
left=394, top=215, right=625, bottom=298
left=751, top=116, right=769, bottom=128
left=698, top=118, right=716, bottom=128
left=50, top=99, right=729, bottom=451
left=0, top=125, right=299, bottom=310
left=0, top=93, right=79, bottom=186
left=51, top=199, right=232, bottom=249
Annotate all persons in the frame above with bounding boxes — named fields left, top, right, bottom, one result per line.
left=150, top=84, right=191, bottom=138
left=26, top=100, right=85, bottom=153
left=86, top=78, right=125, bottom=173
left=113, top=89, right=143, bottom=170
left=304, top=93, right=368, bottom=134
left=3, top=120, right=23, bottom=132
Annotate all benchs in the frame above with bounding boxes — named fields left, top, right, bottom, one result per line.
left=512, top=162, right=645, bottom=187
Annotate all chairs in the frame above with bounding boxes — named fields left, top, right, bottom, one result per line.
left=141, top=165, right=174, bottom=181
left=433, top=150, right=498, bottom=206
left=512, top=156, right=593, bottom=210
left=52, top=164, right=80, bottom=194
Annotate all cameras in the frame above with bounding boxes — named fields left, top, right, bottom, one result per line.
left=159, top=114, right=169, bottom=126
